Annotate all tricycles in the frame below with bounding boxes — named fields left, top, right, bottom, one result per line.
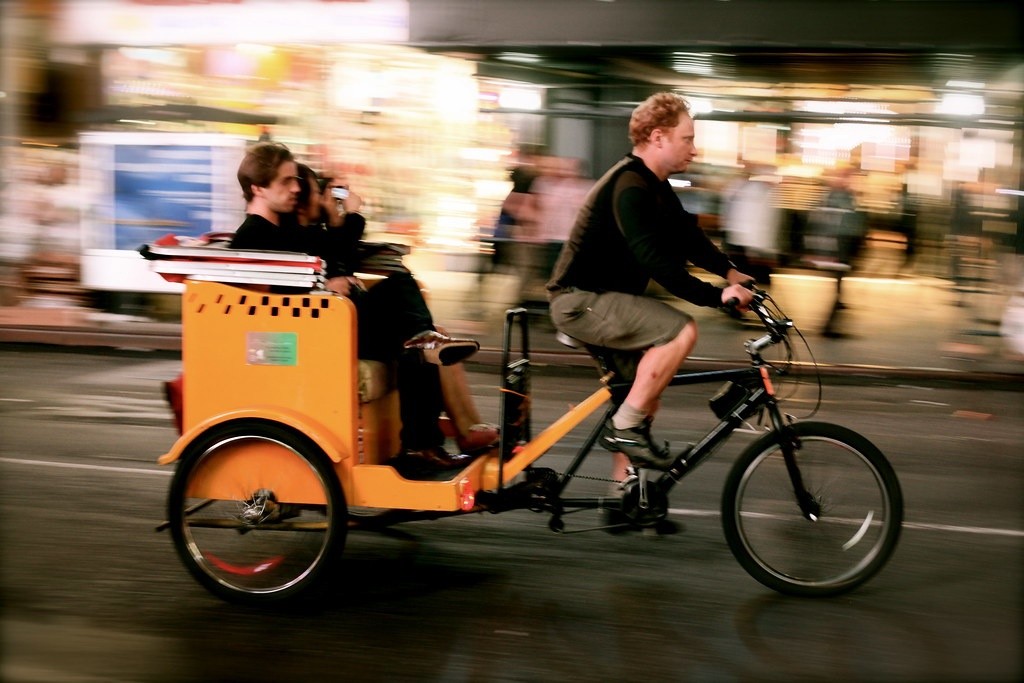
left=155, top=241, right=907, bottom=606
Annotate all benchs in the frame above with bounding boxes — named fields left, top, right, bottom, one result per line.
left=197, top=232, right=403, bottom=405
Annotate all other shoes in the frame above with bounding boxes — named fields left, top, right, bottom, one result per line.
left=458, top=421, right=502, bottom=453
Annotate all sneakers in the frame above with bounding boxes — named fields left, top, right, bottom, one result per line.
left=599, top=415, right=676, bottom=469
left=597, top=506, right=686, bottom=536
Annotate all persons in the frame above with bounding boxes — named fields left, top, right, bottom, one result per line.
left=231, top=142, right=501, bottom=476
left=546, top=90, right=755, bottom=533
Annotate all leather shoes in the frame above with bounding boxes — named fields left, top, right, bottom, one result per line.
left=398, top=445, right=471, bottom=471
left=402, top=330, right=479, bottom=365
